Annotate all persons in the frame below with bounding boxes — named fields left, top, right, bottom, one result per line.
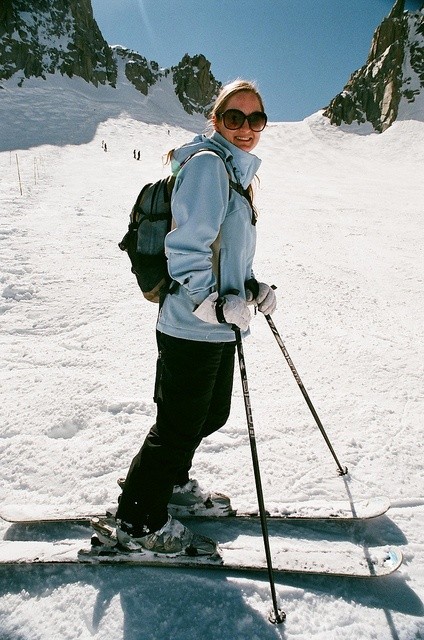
left=92, top=79, right=277, bottom=557
left=136, top=150, right=140, bottom=160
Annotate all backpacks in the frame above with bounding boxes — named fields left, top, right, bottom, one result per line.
left=119, top=148, right=257, bottom=303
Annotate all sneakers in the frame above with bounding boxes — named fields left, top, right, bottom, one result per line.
left=167, top=479, right=230, bottom=511
left=116, top=513, right=214, bottom=557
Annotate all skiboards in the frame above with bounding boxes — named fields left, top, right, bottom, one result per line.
left=0, top=497, right=403, bottom=576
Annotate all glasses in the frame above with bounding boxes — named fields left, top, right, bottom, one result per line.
left=218, top=109, right=267, bottom=132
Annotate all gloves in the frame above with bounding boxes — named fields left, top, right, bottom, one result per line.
left=246, top=282, right=277, bottom=315
left=191, top=289, right=251, bottom=331
